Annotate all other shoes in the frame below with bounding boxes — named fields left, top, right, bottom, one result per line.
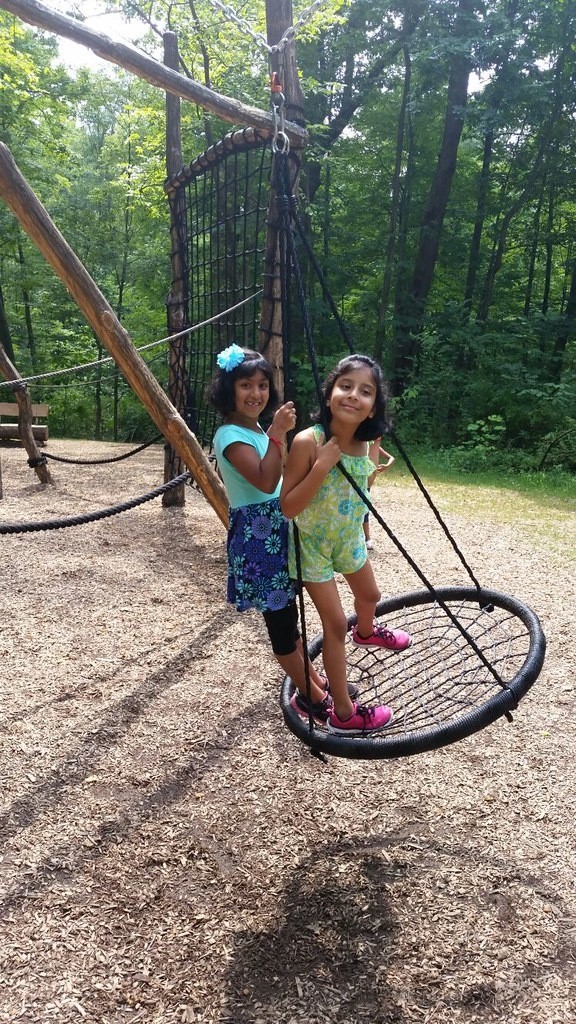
left=366, top=538, right=373, bottom=550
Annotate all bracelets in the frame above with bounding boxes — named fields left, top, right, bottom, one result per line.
left=270, top=436, right=285, bottom=459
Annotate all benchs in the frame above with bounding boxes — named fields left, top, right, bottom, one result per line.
left=0, top=402, right=49, bottom=448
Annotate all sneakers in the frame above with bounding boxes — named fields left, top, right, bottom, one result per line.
left=351, top=623, right=412, bottom=652
left=327, top=701, right=393, bottom=733
left=290, top=674, right=358, bottom=727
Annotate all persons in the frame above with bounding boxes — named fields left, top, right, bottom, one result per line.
left=212, top=343, right=358, bottom=724
left=363, top=447, right=395, bottom=550
left=279, top=354, right=412, bottom=734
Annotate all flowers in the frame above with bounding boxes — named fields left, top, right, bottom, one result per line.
left=217, top=343, right=245, bottom=372
